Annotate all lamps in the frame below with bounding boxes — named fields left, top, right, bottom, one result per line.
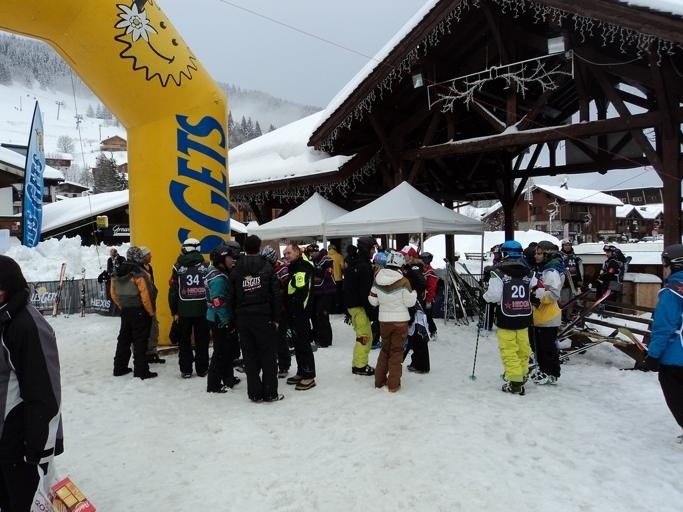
left=411, top=72, right=427, bottom=89
left=546, top=35, right=570, bottom=62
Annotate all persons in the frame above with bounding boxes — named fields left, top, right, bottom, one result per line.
left=327, top=236, right=446, bottom=395
left=0, top=253, right=61, bottom=511
left=634, top=242, right=683, bottom=445
left=595, top=243, right=628, bottom=314
left=104, top=233, right=335, bottom=403
left=481, top=239, right=589, bottom=396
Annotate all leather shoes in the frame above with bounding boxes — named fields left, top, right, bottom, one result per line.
left=148, top=359, right=165, bottom=363
left=295, top=378, right=314, bottom=390
left=127, top=368, right=132, bottom=373
left=286, top=374, right=302, bottom=384
left=134, top=370, right=158, bottom=378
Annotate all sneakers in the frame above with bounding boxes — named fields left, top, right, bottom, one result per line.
left=408, top=367, right=423, bottom=373
left=501, top=366, right=557, bottom=395
left=278, top=369, right=288, bottom=378
left=352, top=367, right=375, bottom=376
left=271, top=394, right=283, bottom=401
left=180, top=358, right=245, bottom=393
left=429, top=331, right=438, bottom=341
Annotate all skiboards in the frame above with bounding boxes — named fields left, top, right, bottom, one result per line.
left=50, top=263, right=67, bottom=318
left=79, top=267, right=85, bottom=317
left=442, top=263, right=493, bottom=330
left=530, top=284, right=658, bottom=373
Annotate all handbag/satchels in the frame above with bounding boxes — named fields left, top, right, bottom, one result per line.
left=169, top=320, right=180, bottom=344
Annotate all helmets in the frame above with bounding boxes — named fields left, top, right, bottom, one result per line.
left=373, top=251, right=388, bottom=267
left=561, top=239, right=572, bottom=244
left=214, top=247, right=234, bottom=257
left=385, top=251, right=406, bottom=268
left=181, top=238, right=200, bottom=253
left=259, top=246, right=277, bottom=265
left=127, top=246, right=144, bottom=265
left=499, top=240, right=522, bottom=257
left=661, top=244, right=682, bottom=271
left=603, top=244, right=616, bottom=251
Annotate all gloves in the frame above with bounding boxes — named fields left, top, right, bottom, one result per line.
left=478, top=295, right=487, bottom=306
left=634, top=359, right=648, bottom=372
left=224, top=323, right=237, bottom=342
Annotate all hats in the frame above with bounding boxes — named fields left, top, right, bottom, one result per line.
left=399, top=245, right=416, bottom=257
left=140, top=247, right=150, bottom=257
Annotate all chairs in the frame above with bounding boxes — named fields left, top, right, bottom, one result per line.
left=593, top=255, right=632, bottom=305
